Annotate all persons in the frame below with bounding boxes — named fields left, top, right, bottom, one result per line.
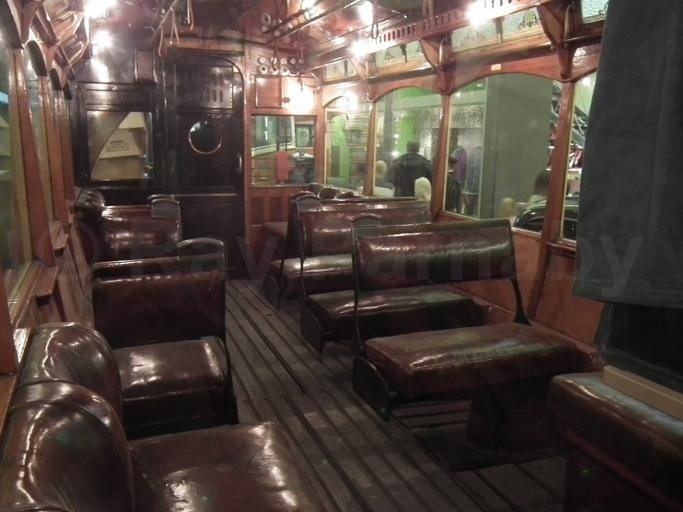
left=529, top=169, right=549, bottom=204
left=388, top=138, right=434, bottom=199
left=349, top=153, right=465, bottom=213
left=448, top=146, right=466, bottom=215
left=464, top=145, right=482, bottom=216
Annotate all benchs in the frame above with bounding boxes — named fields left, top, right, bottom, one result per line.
left=258, top=185, right=579, bottom=471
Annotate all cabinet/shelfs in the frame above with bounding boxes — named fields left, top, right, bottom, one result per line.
left=548, top=372, right=683, bottom=512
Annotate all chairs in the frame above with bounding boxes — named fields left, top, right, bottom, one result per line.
left=0, top=185, right=313, bottom=512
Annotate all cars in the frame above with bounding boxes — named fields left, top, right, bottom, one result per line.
left=516, top=194, right=579, bottom=240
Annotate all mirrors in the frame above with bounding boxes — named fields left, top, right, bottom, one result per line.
left=85, top=109, right=154, bottom=181
left=250, top=114, right=317, bottom=185
